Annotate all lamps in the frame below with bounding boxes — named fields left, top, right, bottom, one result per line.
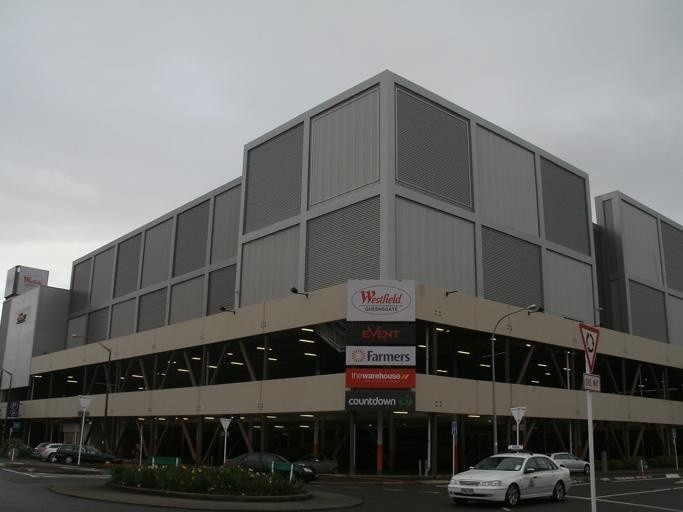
left=290, top=287, right=308, bottom=298
left=220, top=307, right=235, bottom=315
left=528, top=307, right=544, bottom=316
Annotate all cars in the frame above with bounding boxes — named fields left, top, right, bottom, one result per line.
left=223, top=451, right=320, bottom=484
left=291, top=453, right=340, bottom=475
left=546, top=451, right=591, bottom=475
left=446, top=451, right=572, bottom=507
left=31, top=442, right=123, bottom=465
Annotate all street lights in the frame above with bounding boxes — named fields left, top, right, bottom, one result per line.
left=491, top=303, right=537, bottom=465
left=71, top=333, right=112, bottom=445
